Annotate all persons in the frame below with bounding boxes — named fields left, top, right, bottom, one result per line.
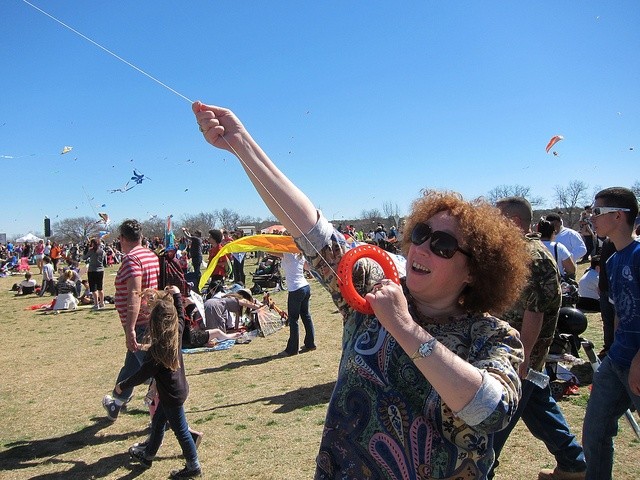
left=105, top=167, right=152, bottom=195
left=557, top=209, right=564, bottom=226
left=64, top=241, right=90, bottom=269
left=44, top=240, right=53, bottom=256
left=143, top=216, right=290, bottom=346
left=631, top=224, right=640, bottom=242
left=596, top=237, right=620, bottom=360
left=82, top=278, right=97, bottom=303
left=101, top=238, right=123, bottom=267
left=128, top=289, right=203, bottom=449
left=578, top=255, right=600, bottom=312
left=278, top=251, right=317, bottom=354
left=580, top=185, right=640, bottom=479
left=53, top=270, right=78, bottom=311
left=33, top=240, right=45, bottom=274
left=101, top=218, right=160, bottom=422
left=0, top=236, right=36, bottom=274
left=494, top=195, right=590, bottom=480
left=38, top=256, right=56, bottom=297
left=51, top=241, right=64, bottom=273
left=545, top=213, right=588, bottom=264
left=83, top=239, right=107, bottom=309
left=581, top=212, right=591, bottom=231
left=95, top=212, right=108, bottom=224
left=536, top=219, right=576, bottom=277
left=337, top=219, right=405, bottom=254
left=252, top=289, right=270, bottom=306
left=62, top=267, right=82, bottom=297
left=18, top=273, right=36, bottom=295
left=116, top=285, right=206, bottom=479
left=579, top=205, right=595, bottom=262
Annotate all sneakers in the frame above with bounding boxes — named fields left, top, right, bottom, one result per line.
left=189, top=429, right=203, bottom=449
left=128, top=447, right=152, bottom=468
left=102, top=395, right=121, bottom=421
left=171, top=467, right=201, bottom=480
left=279, top=351, right=298, bottom=357
left=539, top=466, right=589, bottom=480
left=300, top=345, right=316, bottom=350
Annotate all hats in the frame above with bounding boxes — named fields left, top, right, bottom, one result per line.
left=237, top=289, right=253, bottom=297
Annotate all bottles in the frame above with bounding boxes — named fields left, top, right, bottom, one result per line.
left=524, top=368, right=550, bottom=390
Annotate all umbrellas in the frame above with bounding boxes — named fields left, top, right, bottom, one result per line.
left=83, top=229, right=111, bottom=240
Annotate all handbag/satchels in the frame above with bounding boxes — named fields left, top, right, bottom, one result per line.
left=554, top=242, right=578, bottom=305
left=143, top=377, right=171, bottom=431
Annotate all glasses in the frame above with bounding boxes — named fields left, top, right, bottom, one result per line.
left=592, top=207, right=630, bottom=218
left=410, top=222, right=474, bottom=259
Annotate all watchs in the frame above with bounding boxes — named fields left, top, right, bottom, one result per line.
left=411, top=336, right=440, bottom=362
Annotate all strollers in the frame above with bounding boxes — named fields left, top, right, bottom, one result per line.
left=250, top=256, right=287, bottom=294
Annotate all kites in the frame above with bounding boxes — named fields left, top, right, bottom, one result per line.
left=61, top=145, right=74, bottom=155
